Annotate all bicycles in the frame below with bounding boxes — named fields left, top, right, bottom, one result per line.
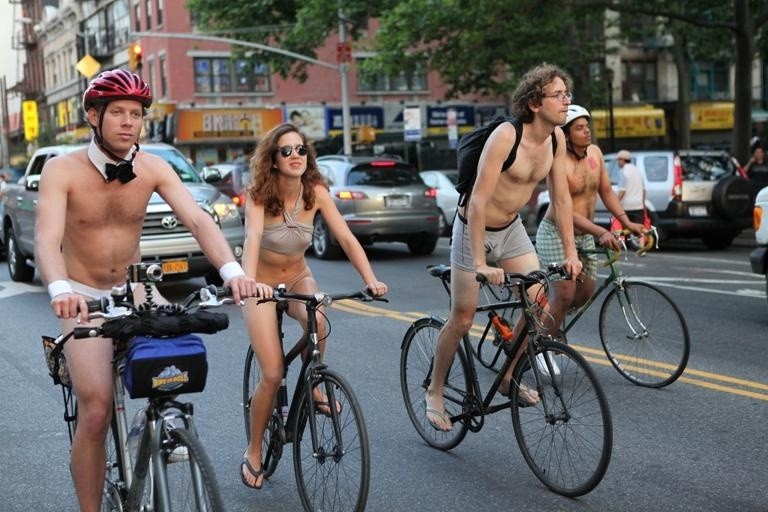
left=450, top=224, right=690, bottom=390
left=400, top=260, right=613, bottom=496
left=42, top=287, right=258, bottom=511
left=243, top=284, right=390, bottom=512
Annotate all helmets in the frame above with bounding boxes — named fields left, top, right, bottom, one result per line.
left=83, top=68, right=153, bottom=113
left=559, top=104, right=591, bottom=127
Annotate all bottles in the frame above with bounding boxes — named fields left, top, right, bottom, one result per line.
left=125, top=406, right=145, bottom=459
left=487, top=310, right=513, bottom=340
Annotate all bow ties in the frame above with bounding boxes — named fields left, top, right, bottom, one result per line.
left=105, top=161, right=137, bottom=185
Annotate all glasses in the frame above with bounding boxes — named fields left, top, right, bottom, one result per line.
left=276, top=145, right=308, bottom=157
left=545, top=92, right=573, bottom=101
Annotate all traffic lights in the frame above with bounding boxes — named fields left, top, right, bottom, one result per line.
left=127, top=43, right=143, bottom=73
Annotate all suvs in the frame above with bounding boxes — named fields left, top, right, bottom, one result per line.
left=535, top=148, right=757, bottom=251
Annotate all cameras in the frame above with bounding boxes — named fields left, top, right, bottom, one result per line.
left=126, top=262, right=164, bottom=283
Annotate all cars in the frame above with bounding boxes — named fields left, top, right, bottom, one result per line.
left=234, top=155, right=439, bottom=260
left=417, top=169, right=460, bottom=236
left=198, top=162, right=252, bottom=207
left=0, top=144, right=246, bottom=297
left=749, top=186, right=768, bottom=302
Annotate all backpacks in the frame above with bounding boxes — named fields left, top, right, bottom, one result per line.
left=455, top=116, right=557, bottom=194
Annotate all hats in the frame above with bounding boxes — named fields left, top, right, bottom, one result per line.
left=613, top=149, right=631, bottom=160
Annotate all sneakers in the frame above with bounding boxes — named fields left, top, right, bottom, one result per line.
left=535, top=351, right=560, bottom=376
left=163, top=439, right=189, bottom=462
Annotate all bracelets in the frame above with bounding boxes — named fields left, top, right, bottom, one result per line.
left=219, top=262, right=244, bottom=282
left=48, top=280, right=73, bottom=298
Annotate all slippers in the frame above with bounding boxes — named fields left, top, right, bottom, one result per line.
left=314, top=400, right=343, bottom=418
left=423, top=399, right=452, bottom=431
left=498, top=383, right=540, bottom=408
left=240, top=449, right=263, bottom=489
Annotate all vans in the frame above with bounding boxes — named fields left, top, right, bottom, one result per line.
left=1, top=153, right=31, bottom=185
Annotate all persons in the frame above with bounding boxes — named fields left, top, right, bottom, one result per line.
left=425, top=63, right=584, bottom=433
left=533, top=101, right=649, bottom=373
left=611, top=148, right=650, bottom=256
left=33, top=70, right=257, bottom=512
left=238, top=123, right=390, bottom=491
left=747, top=147, right=767, bottom=181
left=288, top=111, right=322, bottom=137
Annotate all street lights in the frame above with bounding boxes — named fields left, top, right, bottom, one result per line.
left=15, top=17, right=96, bottom=143
left=602, top=67, right=615, bottom=153
